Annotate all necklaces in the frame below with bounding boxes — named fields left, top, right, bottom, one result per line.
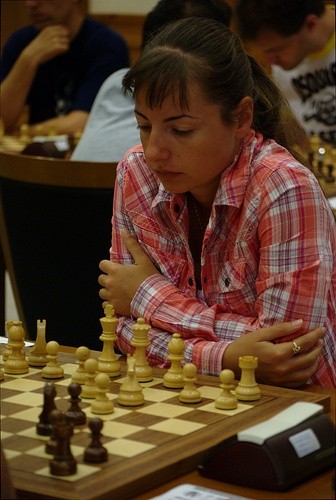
left=193, top=201, right=205, bottom=234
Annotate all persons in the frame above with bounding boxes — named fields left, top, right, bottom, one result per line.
left=98, top=16, right=336, bottom=397
left=1, top=0, right=336, bottom=164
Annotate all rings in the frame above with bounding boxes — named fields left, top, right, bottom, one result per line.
left=289, top=340, right=301, bottom=355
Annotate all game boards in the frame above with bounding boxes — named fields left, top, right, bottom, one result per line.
left=295, top=136, right=336, bottom=197
left=0, top=134, right=80, bottom=159
left=0, top=343, right=277, bottom=483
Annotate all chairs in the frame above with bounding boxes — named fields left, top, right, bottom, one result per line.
left=0, top=148, right=124, bottom=356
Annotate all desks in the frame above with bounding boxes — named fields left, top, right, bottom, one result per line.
left=129, top=384, right=336, bottom=500
